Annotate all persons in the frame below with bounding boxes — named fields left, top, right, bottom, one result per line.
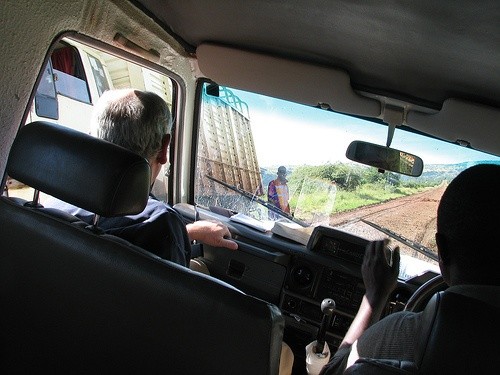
left=40, top=87, right=239, bottom=288
left=316, top=163, right=500, bottom=374
left=267, top=166, right=293, bottom=222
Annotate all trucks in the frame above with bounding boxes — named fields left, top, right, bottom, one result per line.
left=12, top=38, right=267, bottom=221
